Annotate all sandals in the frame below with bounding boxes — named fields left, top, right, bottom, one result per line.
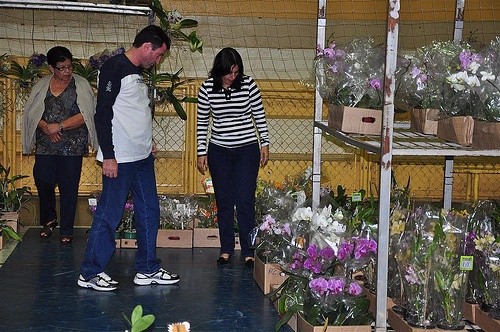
left=60, top=234, right=74, bottom=244
left=40, top=217, right=59, bottom=239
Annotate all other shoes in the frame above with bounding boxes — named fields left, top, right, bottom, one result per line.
left=245, top=257, right=255, bottom=268
left=219, top=252, right=232, bottom=262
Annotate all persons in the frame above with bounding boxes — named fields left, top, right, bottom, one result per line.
left=19, top=46, right=95, bottom=243
left=196, top=47, right=271, bottom=268
left=76, top=23, right=182, bottom=291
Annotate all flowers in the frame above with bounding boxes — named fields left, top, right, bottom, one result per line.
left=89, top=179, right=218, bottom=238
left=120, top=305, right=191, bottom=332
left=256, top=33, right=500, bottom=332
left=0, top=0, right=204, bottom=122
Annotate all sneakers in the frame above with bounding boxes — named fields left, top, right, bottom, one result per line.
left=133, top=267, right=180, bottom=285
left=77, top=271, right=119, bottom=291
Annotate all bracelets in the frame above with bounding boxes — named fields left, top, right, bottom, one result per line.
left=58, top=122, right=65, bottom=132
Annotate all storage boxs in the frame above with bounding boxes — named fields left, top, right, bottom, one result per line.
left=437, top=116, right=473, bottom=145
left=327, top=103, right=382, bottom=135
left=410, top=107, right=439, bottom=134
left=471, top=120, right=500, bottom=151
left=107, top=228, right=500, bottom=332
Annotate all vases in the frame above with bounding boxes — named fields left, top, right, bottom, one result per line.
left=125, top=231, right=137, bottom=239
left=114, top=231, right=120, bottom=239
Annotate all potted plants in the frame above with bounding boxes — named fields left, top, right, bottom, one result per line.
left=0, top=164, right=37, bottom=252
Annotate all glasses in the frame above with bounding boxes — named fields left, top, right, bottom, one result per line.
left=53, top=64, right=73, bottom=71
